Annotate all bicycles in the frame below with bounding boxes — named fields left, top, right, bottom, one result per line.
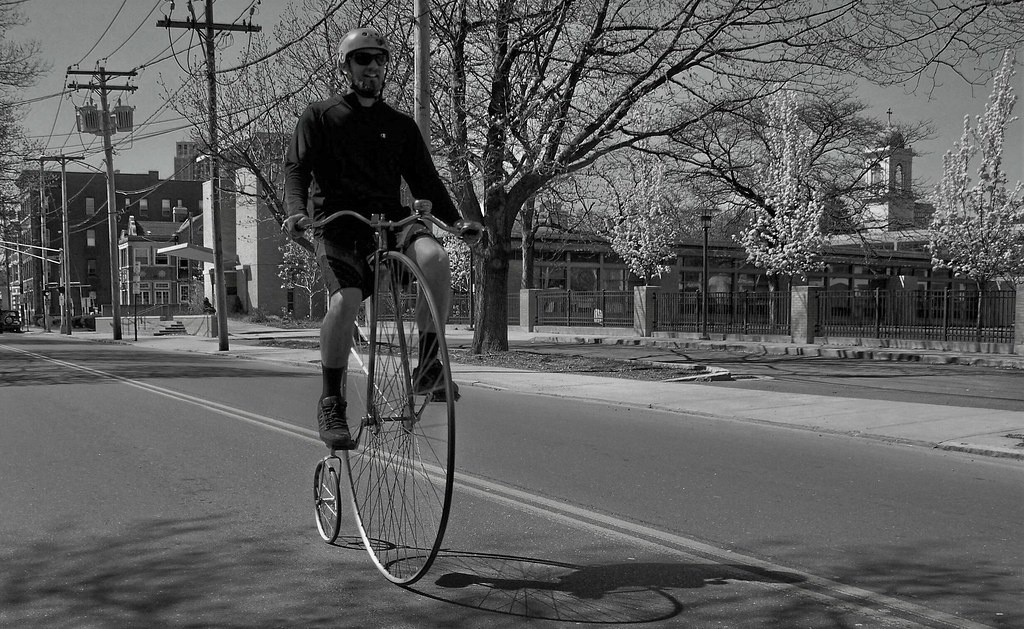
left=291, top=197, right=480, bottom=587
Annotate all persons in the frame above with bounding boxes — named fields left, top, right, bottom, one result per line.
left=280, top=27, right=482, bottom=451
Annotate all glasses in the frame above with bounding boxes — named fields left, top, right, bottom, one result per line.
left=348, top=51, right=388, bottom=66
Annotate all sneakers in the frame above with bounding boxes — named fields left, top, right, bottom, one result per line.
left=316, top=395, right=351, bottom=446
left=412, top=359, right=459, bottom=399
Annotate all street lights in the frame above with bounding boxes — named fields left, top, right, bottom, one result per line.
left=698, top=205, right=714, bottom=340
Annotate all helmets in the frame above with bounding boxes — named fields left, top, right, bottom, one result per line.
left=336, top=28, right=392, bottom=68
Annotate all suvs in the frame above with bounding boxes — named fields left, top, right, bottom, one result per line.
left=0, top=309, right=22, bottom=333
